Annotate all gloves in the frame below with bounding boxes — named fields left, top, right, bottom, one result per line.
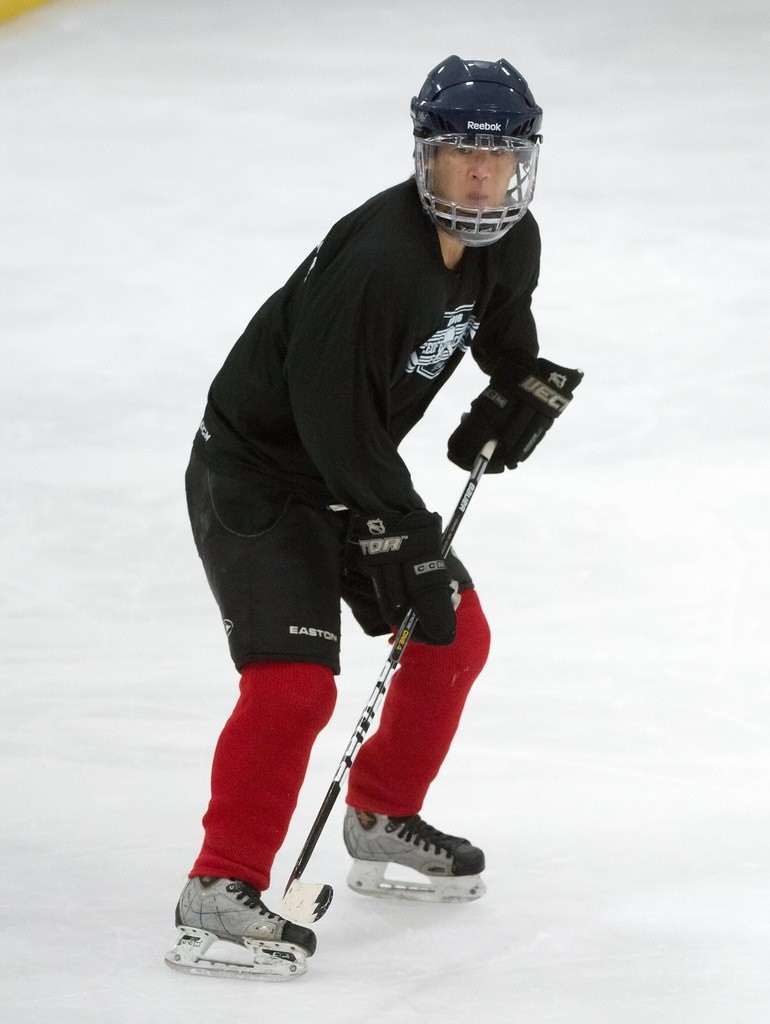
left=362, top=507, right=460, bottom=647
left=446, top=357, right=584, bottom=474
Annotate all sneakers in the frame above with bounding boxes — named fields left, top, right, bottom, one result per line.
left=343, top=805, right=485, bottom=903
left=164, top=875, right=317, bottom=982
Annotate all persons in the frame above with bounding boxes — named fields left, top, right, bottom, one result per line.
left=165, top=55, right=583, bottom=982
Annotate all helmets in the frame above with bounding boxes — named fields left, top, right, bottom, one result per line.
left=409, top=55, right=542, bottom=247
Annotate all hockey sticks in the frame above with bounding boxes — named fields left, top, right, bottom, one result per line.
left=280, top=433, right=500, bottom=924
